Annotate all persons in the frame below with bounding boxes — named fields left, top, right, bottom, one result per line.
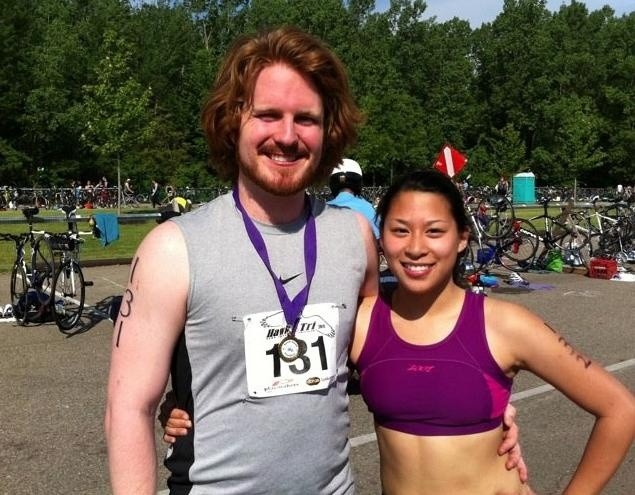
left=103, top=22, right=528, bottom=495
left=498, top=176, right=508, bottom=212
left=150, top=178, right=163, bottom=208
left=324, top=158, right=381, bottom=395
left=158, top=171, right=634, bottom=495
left=74, top=176, right=135, bottom=209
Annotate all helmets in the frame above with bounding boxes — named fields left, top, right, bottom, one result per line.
left=327, top=155, right=364, bottom=179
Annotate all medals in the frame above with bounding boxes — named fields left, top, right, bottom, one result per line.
left=277, top=328, right=304, bottom=362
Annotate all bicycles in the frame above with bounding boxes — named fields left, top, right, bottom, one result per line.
left=456, top=173, right=634, bottom=280
left=0, top=205, right=93, bottom=330
